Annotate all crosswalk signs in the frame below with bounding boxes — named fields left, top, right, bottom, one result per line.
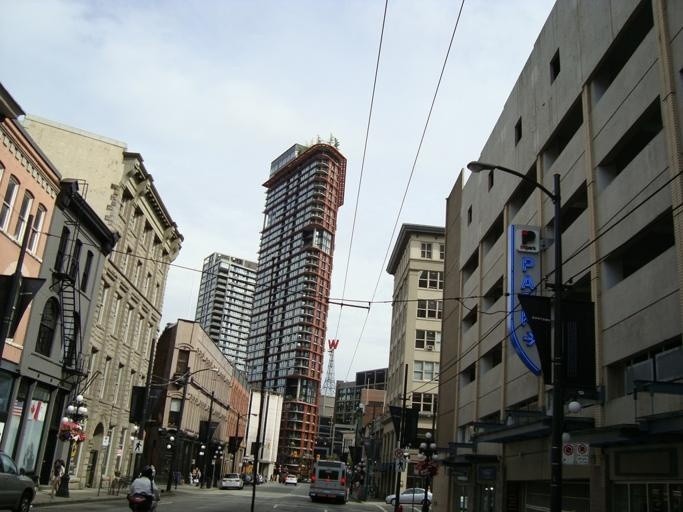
left=395, top=458, right=407, bottom=473
left=132, top=440, right=144, bottom=454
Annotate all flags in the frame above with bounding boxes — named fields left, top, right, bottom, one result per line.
left=12, top=399, right=24, bottom=417
left=28, top=400, right=48, bottom=423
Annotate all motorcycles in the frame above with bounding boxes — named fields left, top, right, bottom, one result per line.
left=124, top=490, right=160, bottom=512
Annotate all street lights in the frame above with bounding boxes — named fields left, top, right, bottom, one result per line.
left=354, top=458, right=367, bottom=500
left=167, top=366, right=219, bottom=493
left=467, top=162, right=563, bottom=512
left=417, top=432, right=439, bottom=511
left=231, top=412, right=257, bottom=473
left=56, top=394, right=88, bottom=497
left=214, top=447, right=223, bottom=487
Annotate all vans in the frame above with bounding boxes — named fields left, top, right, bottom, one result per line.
left=309, top=459, right=347, bottom=504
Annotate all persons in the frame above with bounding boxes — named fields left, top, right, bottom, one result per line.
left=127, top=463, right=160, bottom=512
left=51, top=458, right=66, bottom=495
left=135, top=441, right=142, bottom=451
left=196, top=470, right=199, bottom=486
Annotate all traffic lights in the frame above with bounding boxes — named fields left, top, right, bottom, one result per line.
left=198, top=442, right=207, bottom=457
left=165, top=429, right=176, bottom=455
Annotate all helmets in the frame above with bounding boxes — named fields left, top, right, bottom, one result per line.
left=143, top=464, right=156, bottom=477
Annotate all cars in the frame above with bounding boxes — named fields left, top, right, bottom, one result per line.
left=385, top=487, right=433, bottom=506
left=0, top=454, right=36, bottom=512
left=219, top=473, right=254, bottom=490
left=285, top=475, right=298, bottom=485
